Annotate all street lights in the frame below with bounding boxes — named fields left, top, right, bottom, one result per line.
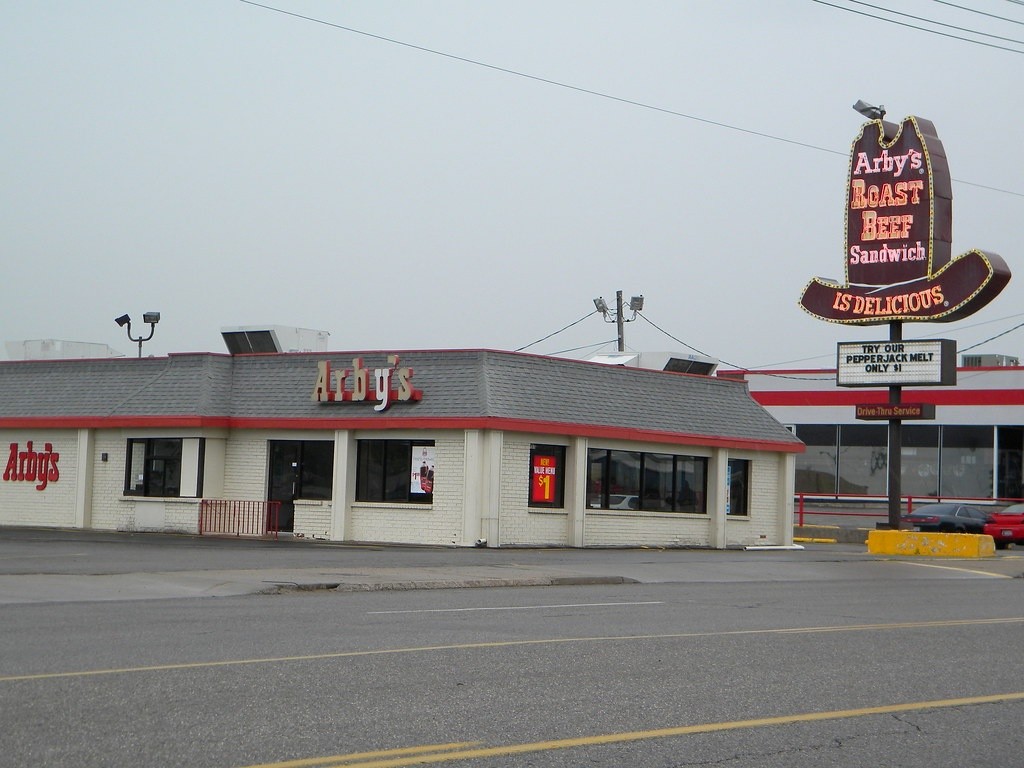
left=595, top=291, right=646, bottom=352
left=114, top=313, right=161, bottom=357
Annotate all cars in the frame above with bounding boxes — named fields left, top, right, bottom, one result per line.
left=900, top=503, right=992, bottom=534
left=982, top=503, right=1024, bottom=552
left=589, top=494, right=642, bottom=511
